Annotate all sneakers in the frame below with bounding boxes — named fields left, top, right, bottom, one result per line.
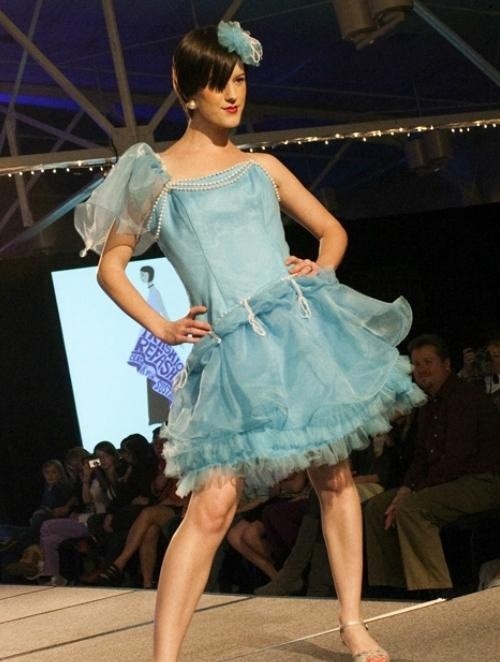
left=253, top=577, right=304, bottom=595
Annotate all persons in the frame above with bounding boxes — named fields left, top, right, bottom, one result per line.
left=72, top=21, right=427, bottom=662
left=1, top=332, right=500, bottom=597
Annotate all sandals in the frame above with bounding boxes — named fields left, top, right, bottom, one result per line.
left=338, top=614, right=390, bottom=662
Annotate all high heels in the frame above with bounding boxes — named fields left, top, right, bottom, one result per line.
left=97, top=563, right=122, bottom=586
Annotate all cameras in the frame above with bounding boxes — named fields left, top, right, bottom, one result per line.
left=88, top=457, right=101, bottom=468
left=470, top=342, right=492, bottom=366
